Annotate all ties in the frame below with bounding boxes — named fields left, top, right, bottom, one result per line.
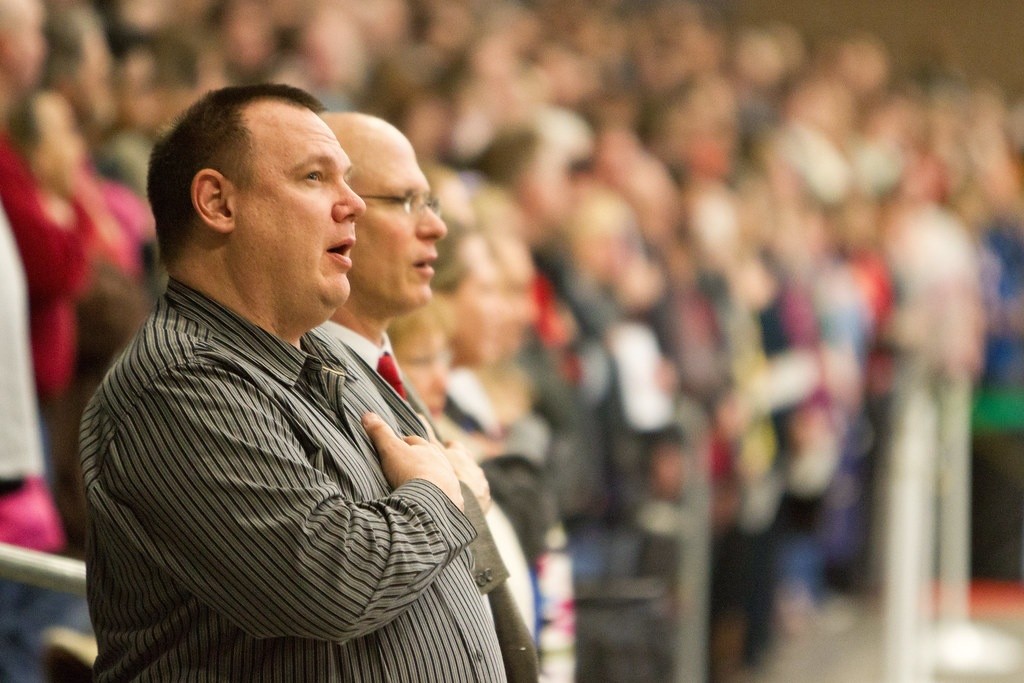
left=378, top=354, right=408, bottom=401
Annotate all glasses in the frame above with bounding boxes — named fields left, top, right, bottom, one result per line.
left=358, top=189, right=443, bottom=219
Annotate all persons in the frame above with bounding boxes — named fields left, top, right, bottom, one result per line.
left=1, top=0, right=1024, bottom=683
left=80, top=84, right=508, bottom=683
